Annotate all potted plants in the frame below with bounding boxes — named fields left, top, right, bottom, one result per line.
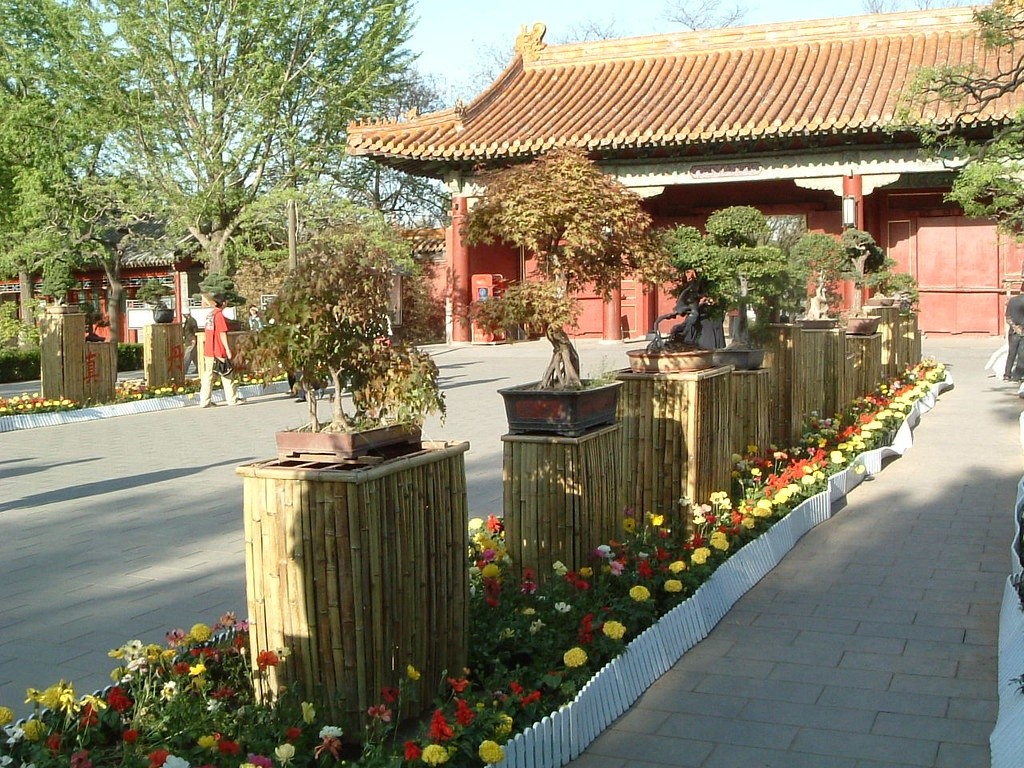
left=41, top=255, right=80, bottom=313
left=137, top=280, right=175, bottom=323
left=202, top=222, right=448, bottom=463
left=462, top=160, right=920, bottom=439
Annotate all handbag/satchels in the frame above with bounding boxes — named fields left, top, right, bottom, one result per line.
left=212, top=357, right=234, bottom=377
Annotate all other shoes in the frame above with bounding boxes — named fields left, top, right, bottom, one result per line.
left=318, top=392, right=324, bottom=400
left=192, top=370, right=198, bottom=374
left=296, top=399, right=306, bottom=402
left=229, top=397, right=246, bottom=405
left=200, top=402, right=217, bottom=408
left=1004, top=376, right=1011, bottom=380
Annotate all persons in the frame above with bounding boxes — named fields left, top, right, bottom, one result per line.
left=247, top=304, right=265, bottom=331
left=286, top=372, right=326, bottom=402
left=674, top=264, right=726, bottom=349
left=179, top=308, right=200, bottom=375
left=199, top=293, right=246, bottom=408
left=1003, top=281, right=1024, bottom=382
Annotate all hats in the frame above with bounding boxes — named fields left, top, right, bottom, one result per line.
left=182, top=308, right=191, bottom=314
left=250, top=306, right=258, bottom=312
